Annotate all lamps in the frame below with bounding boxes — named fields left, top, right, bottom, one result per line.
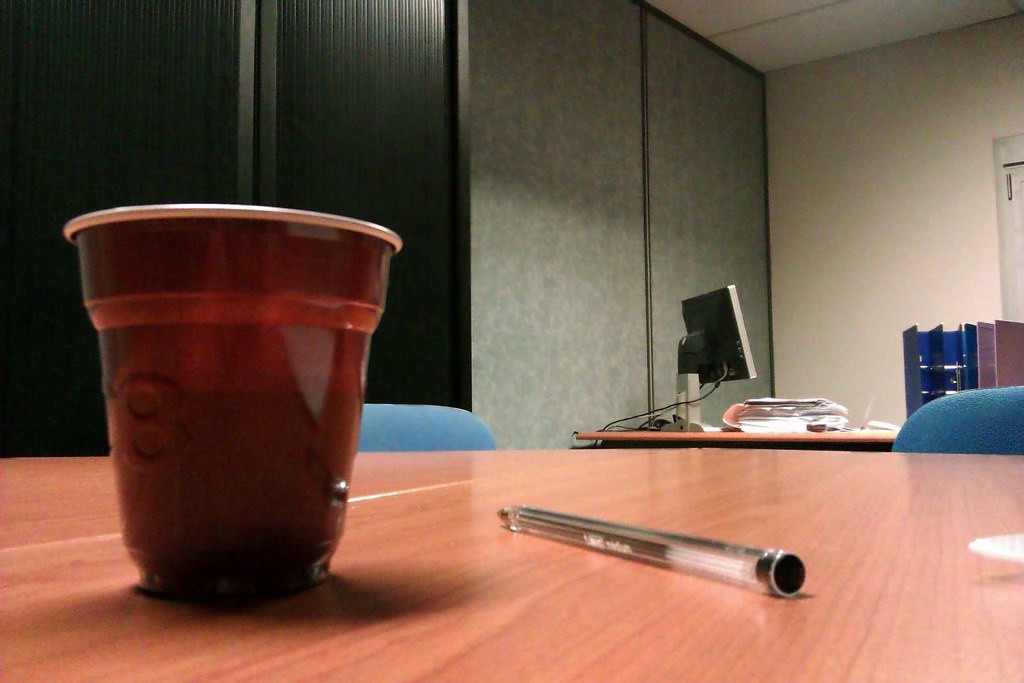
left=675, top=282, right=757, bottom=432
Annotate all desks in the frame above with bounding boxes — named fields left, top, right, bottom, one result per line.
left=0, top=445, right=1024, bottom=683
left=574, top=421, right=905, bottom=443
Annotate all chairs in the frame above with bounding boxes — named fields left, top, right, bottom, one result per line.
left=359, top=403, right=496, bottom=453
left=892, top=386, right=1024, bottom=455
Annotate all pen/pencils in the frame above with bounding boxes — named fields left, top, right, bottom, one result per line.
left=497, top=504, right=807, bottom=601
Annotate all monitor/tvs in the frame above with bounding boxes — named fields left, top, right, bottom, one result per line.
left=661, top=285, right=758, bottom=433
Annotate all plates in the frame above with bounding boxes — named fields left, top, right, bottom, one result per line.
left=969, top=534, right=1024, bottom=562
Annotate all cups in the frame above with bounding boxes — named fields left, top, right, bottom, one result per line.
left=65, top=202, right=404, bottom=598
